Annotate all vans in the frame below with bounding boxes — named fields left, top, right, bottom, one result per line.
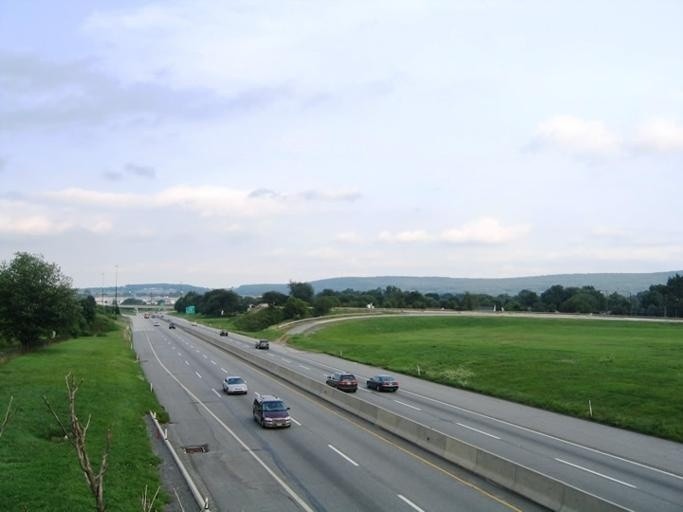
left=253, top=394, right=291, bottom=428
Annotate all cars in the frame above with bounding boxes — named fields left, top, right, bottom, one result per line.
left=222, top=376, right=248, bottom=394
left=326, top=371, right=358, bottom=392
left=255, top=340, right=269, bottom=349
left=367, top=375, right=399, bottom=392
left=220, top=331, right=228, bottom=336
left=144, top=313, right=198, bottom=329
